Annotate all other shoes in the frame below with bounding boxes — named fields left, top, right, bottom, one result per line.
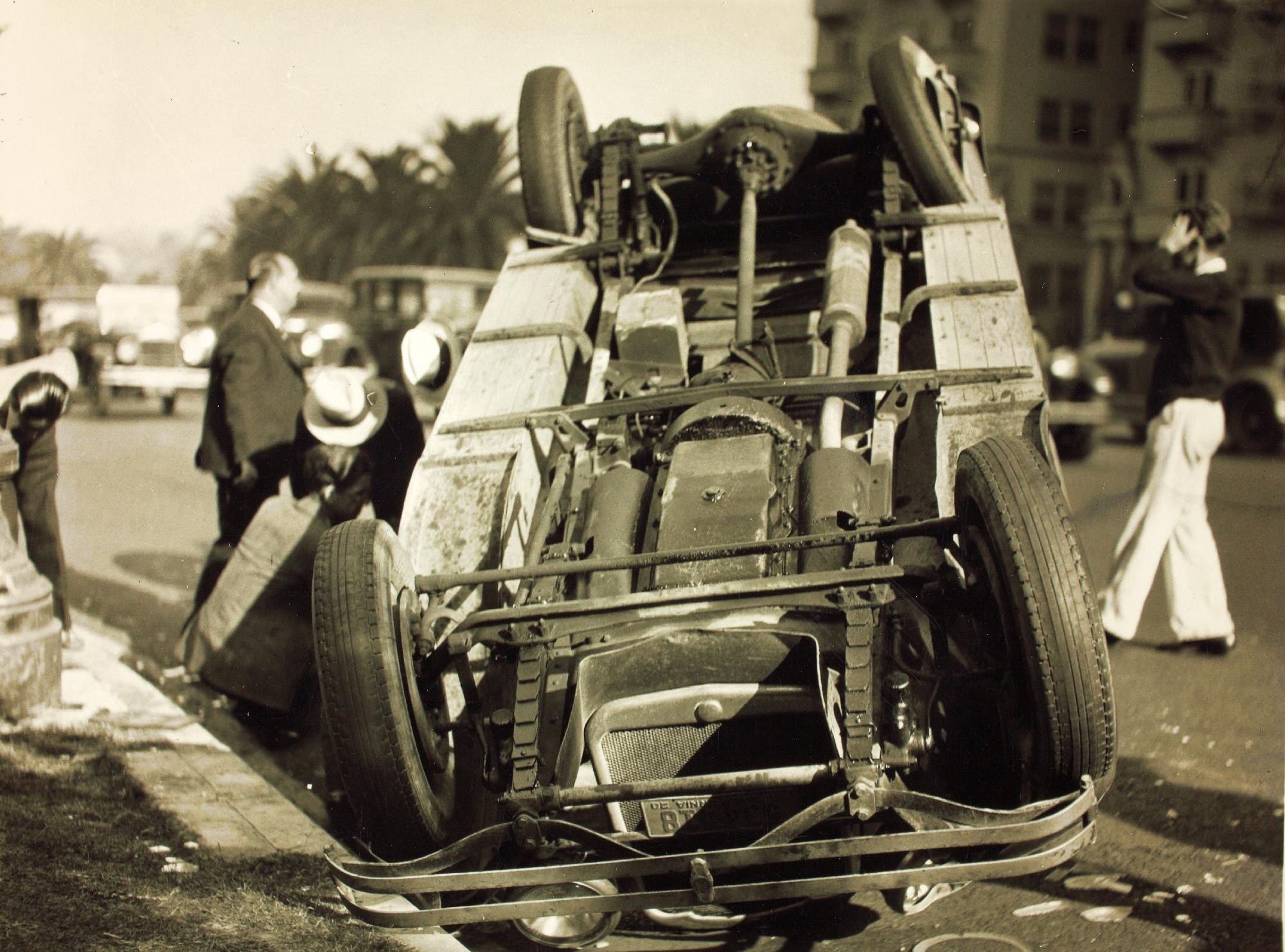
left=1105, top=630, right=1122, bottom=651
left=1157, top=632, right=1237, bottom=655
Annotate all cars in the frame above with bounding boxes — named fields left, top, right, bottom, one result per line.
left=307, top=31, right=1115, bottom=951
left=1, top=264, right=497, bottom=417
left=1034, top=330, right=1114, bottom=461
left=1221, top=291, right=1284, bottom=454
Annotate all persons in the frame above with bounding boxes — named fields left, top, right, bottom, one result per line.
left=175, top=444, right=378, bottom=736
left=1100, top=201, right=1242, bottom=652
left=302, top=365, right=425, bottom=534
left=192, top=250, right=307, bottom=610
left=0, top=348, right=79, bottom=648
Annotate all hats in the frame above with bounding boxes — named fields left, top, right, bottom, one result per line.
left=400, top=317, right=459, bottom=407
left=305, top=366, right=388, bottom=447
